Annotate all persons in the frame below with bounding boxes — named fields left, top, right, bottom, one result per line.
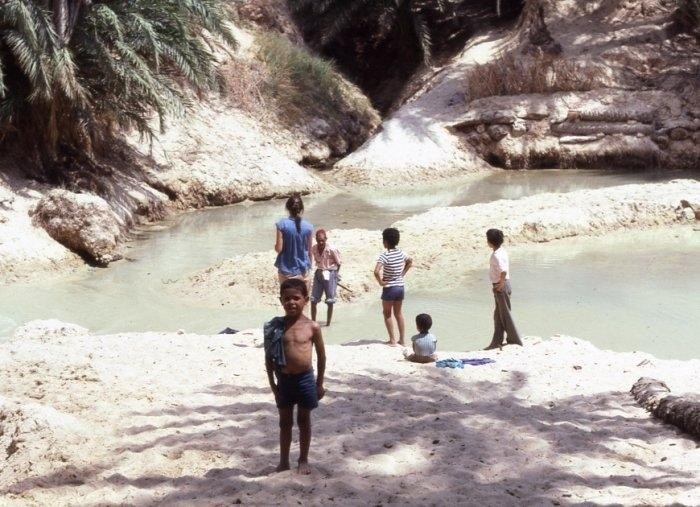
left=310, top=229, right=343, bottom=327
left=483, top=228, right=523, bottom=351
left=402, top=314, right=438, bottom=363
left=274, top=193, right=313, bottom=306
left=264, top=279, right=326, bottom=476
left=373, top=228, right=412, bottom=346
